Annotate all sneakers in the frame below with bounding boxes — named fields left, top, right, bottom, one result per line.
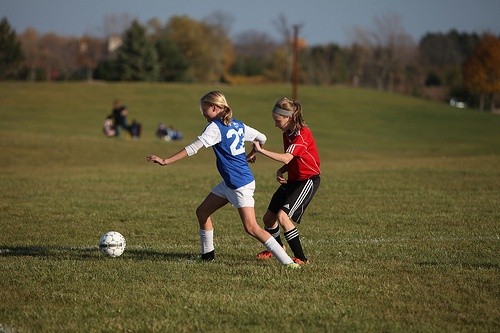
left=286, top=264, right=302, bottom=270
left=292, top=258, right=310, bottom=266
left=255, top=244, right=286, bottom=259
left=196, top=250, right=215, bottom=262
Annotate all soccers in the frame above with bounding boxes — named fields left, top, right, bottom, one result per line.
left=98, top=231, right=126, bottom=258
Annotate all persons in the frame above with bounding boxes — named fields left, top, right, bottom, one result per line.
left=103, top=99, right=182, bottom=142
left=146, top=91, right=301, bottom=269
left=251, top=97, right=321, bottom=266
